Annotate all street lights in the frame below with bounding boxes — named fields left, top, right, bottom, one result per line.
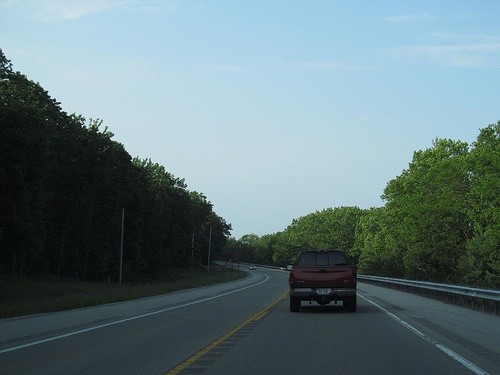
left=207, top=225, right=230, bottom=274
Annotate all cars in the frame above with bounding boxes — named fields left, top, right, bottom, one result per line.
left=249, top=264, right=256, bottom=270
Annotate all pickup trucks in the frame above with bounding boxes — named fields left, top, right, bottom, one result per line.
left=285, top=249, right=358, bottom=313
